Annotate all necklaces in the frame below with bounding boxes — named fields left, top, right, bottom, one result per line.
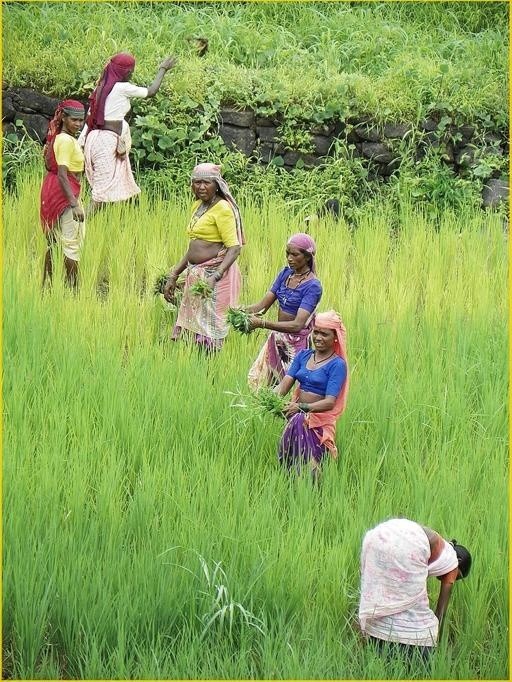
left=188, top=194, right=219, bottom=231
left=310, top=350, right=336, bottom=369
left=281, top=268, right=312, bottom=305
left=62, top=128, right=77, bottom=138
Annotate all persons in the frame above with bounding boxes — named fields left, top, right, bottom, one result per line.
left=75, top=51, right=180, bottom=220
left=162, top=160, right=246, bottom=360
left=358, top=518, right=471, bottom=669
left=261, top=308, right=349, bottom=489
left=225, top=233, right=324, bottom=399
left=37, top=98, right=89, bottom=297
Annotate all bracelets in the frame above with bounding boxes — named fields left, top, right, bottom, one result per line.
left=70, top=201, right=78, bottom=209
left=261, top=320, right=267, bottom=328
left=299, top=402, right=310, bottom=414
left=169, top=271, right=180, bottom=278
left=212, top=267, right=225, bottom=282
left=158, top=66, right=168, bottom=72
